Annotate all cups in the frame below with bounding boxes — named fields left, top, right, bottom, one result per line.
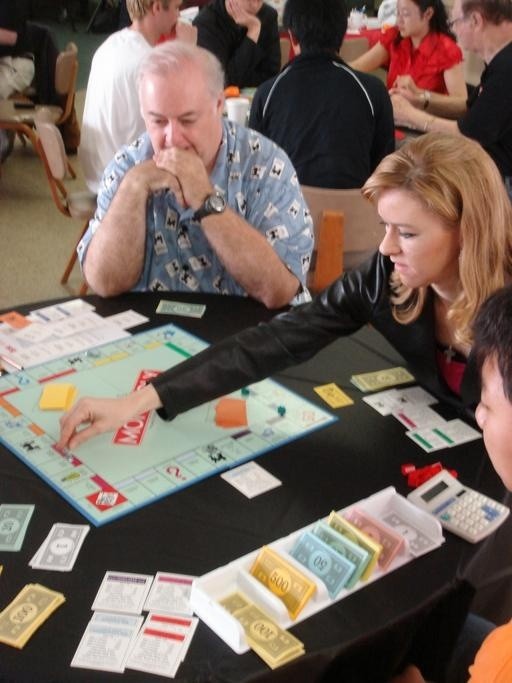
left=225, top=97, right=249, bottom=127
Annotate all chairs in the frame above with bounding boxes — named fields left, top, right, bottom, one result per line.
left=295, top=183, right=389, bottom=292
left=278, top=35, right=486, bottom=89
left=33, top=121, right=102, bottom=296
left=2, top=41, right=82, bottom=178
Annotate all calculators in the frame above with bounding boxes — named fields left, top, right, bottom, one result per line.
left=407, top=469, right=510, bottom=544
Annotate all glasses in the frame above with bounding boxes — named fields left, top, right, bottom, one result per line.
left=444, top=15, right=471, bottom=28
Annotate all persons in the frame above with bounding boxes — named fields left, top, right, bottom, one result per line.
left=0, top=1, right=512, bottom=271
left=58, top=130, right=511, bottom=453
left=74, top=40, right=316, bottom=308
left=390, top=284, right=511, bottom=682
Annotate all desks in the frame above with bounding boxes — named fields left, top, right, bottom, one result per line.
left=277, top=15, right=389, bottom=69
left=223, top=87, right=417, bottom=151
left=1, top=291, right=512, bottom=683
